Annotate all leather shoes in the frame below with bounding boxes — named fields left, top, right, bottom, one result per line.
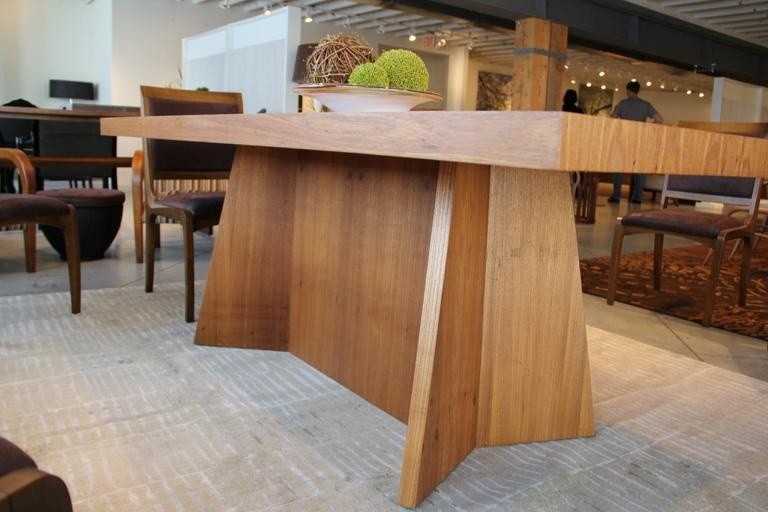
left=607, top=197, right=643, bottom=204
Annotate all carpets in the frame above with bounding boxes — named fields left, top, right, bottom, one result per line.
left=579, top=239, right=767, bottom=342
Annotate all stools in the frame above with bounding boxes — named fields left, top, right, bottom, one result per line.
left=1, top=439, right=71, bottom=512
left=32, top=186, right=124, bottom=262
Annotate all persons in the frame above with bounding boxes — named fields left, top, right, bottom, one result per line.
left=560, top=86, right=589, bottom=199
left=606, top=80, right=665, bottom=205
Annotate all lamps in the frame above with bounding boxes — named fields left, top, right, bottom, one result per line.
left=221, top=1, right=481, bottom=51
left=564, top=57, right=712, bottom=100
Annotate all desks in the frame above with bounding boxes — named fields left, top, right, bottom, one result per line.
left=1, top=105, right=141, bottom=186
left=99, top=108, right=768, bottom=509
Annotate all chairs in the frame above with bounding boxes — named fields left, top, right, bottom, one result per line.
left=0, top=148, right=83, bottom=316
left=140, top=85, right=246, bottom=321
left=31, top=115, right=118, bottom=193
left=703, top=176, right=767, bottom=265
left=603, top=120, right=768, bottom=327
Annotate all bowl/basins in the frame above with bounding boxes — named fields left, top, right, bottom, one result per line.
left=292, top=82, right=445, bottom=115
